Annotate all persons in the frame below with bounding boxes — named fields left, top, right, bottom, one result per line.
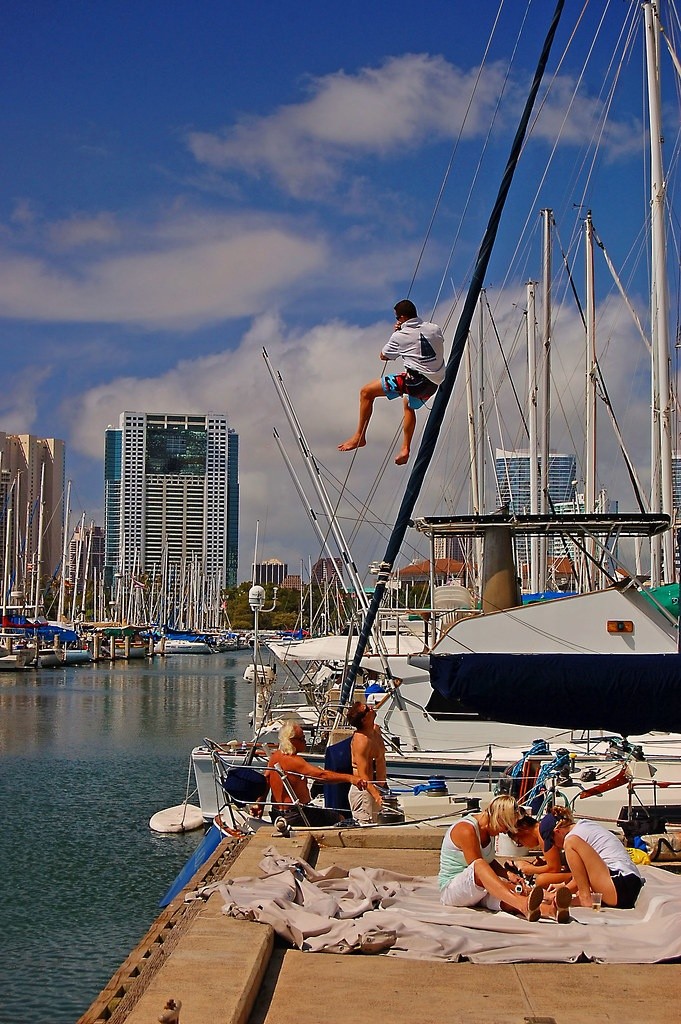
left=249, top=720, right=368, bottom=827
left=348, top=699, right=386, bottom=827
left=539, top=805, right=646, bottom=909
left=339, top=300, right=446, bottom=465
left=505, top=806, right=573, bottom=888
left=365, top=678, right=404, bottom=702
left=438, top=794, right=572, bottom=924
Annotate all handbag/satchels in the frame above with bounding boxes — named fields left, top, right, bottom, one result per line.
left=635, top=833, right=681, bottom=861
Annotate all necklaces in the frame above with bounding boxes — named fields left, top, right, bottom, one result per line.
left=562, top=831, right=570, bottom=853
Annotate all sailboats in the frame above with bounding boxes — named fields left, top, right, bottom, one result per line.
left=1, top=462, right=238, bottom=670
left=192, top=0, right=681, bottom=836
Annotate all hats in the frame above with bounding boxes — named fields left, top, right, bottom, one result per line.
left=539, top=813, right=560, bottom=852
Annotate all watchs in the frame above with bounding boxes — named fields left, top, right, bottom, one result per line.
left=515, top=885, right=522, bottom=895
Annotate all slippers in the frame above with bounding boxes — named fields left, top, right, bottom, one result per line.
left=553, top=887, right=572, bottom=924
left=528, top=886, right=543, bottom=922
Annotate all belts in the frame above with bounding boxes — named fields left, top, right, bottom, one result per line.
left=272, top=808, right=295, bottom=814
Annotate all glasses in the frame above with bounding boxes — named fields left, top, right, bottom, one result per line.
left=549, top=832, right=556, bottom=844
left=362, top=704, right=370, bottom=717
left=396, top=315, right=402, bottom=320
left=291, top=734, right=305, bottom=742
left=517, top=838, right=523, bottom=847
